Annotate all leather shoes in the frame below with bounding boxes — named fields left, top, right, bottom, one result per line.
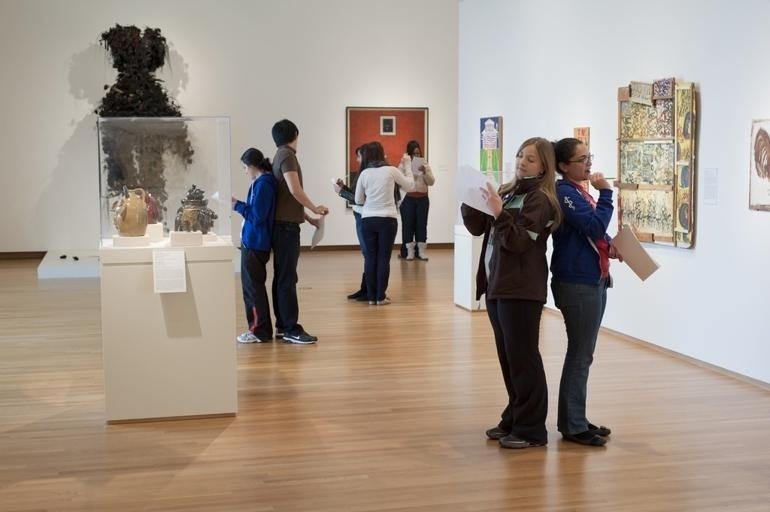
left=589, top=425, right=611, bottom=436
left=566, top=433, right=607, bottom=446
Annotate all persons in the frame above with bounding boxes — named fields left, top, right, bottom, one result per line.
left=334, top=143, right=368, bottom=301
left=231, top=148, right=277, bottom=343
left=461, top=137, right=563, bottom=449
left=397, top=141, right=434, bottom=260
left=355, top=142, right=415, bottom=305
left=550, top=138, right=623, bottom=446
left=272, top=120, right=328, bottom=344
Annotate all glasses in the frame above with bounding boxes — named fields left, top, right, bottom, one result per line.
left=569, top=153, right=595, bottom=165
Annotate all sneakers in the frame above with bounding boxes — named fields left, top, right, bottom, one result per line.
left=486, top=425, right=509, bottom=439
left=236, top=331, right=264, bottom=344
left=282, top=328, right=318, bottom=345
left=368, top=298, right=392, bottom=305
left=275, top=327, right=285, bottom=339
left=498, top=434, right=548, bottom=449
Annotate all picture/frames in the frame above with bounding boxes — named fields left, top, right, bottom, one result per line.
left=379, top=115, right=396, bottom=136
left=346, top=106, right=429, bottom=209
left=749, top=118, right=770, bottom=211
left=480, top=117, right=503, bottom=184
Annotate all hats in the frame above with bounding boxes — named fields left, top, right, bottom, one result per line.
left=271, top=119, right=299, bottom=148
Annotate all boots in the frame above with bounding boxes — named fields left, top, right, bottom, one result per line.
left=405, top=241, right=417, bottom=261
left=417, top=241, right=429, bottom=261
left=347, top=271, right=372, bottom=302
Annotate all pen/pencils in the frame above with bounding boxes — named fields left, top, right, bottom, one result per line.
left=604, top=178, right=616, bottom=179
left=339, top=175, right=349, bottom=181
